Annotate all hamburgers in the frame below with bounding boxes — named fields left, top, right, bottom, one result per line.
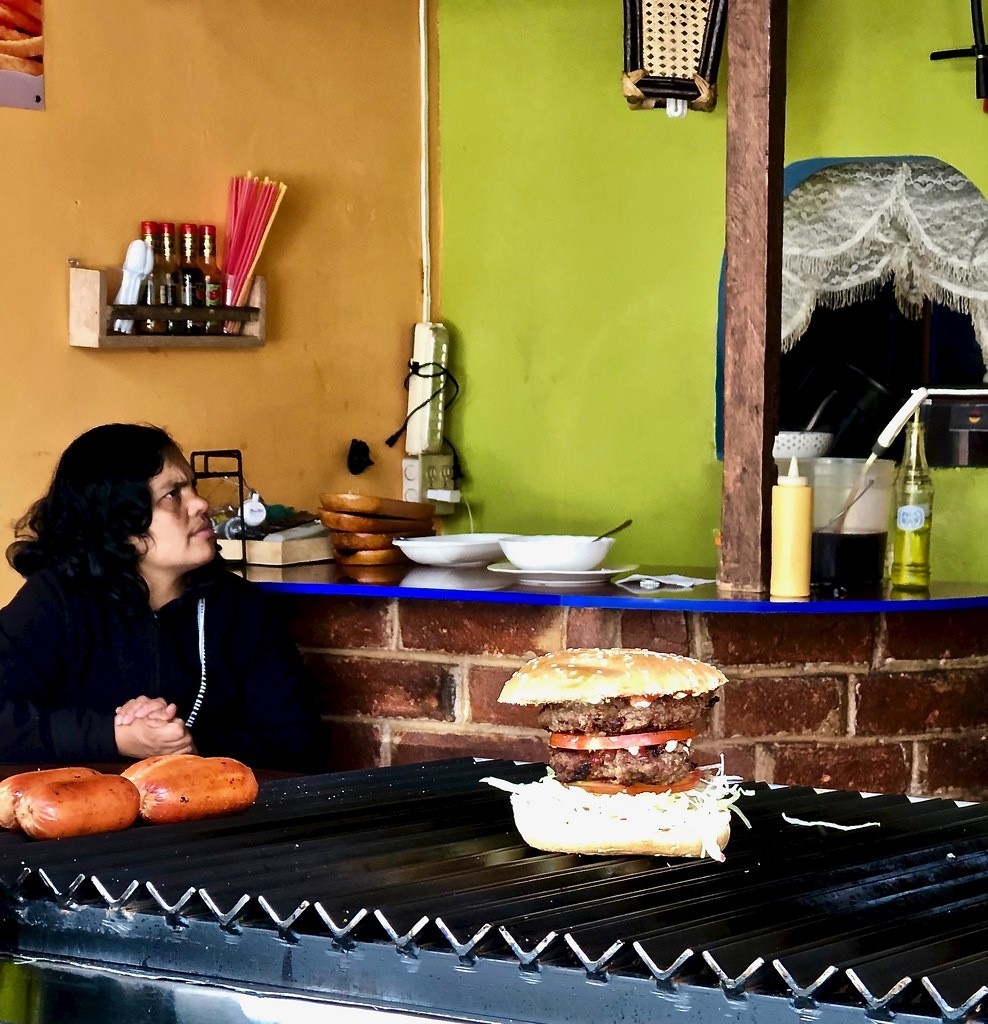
left=495, top=648, right=753, bottom=861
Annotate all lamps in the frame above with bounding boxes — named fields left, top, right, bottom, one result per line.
left=623, top=0, right=728, bottom=119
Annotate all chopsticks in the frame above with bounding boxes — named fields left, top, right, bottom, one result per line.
left=222, top=170, right=292, bottom=333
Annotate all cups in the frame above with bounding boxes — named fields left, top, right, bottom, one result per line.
left=222, top=273, right=253, bottom=335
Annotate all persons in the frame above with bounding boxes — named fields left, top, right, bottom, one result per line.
left=0, top=424, right=330, bottom=771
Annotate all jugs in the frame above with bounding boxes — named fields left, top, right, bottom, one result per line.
left=814, top=458, right=897, bottom=594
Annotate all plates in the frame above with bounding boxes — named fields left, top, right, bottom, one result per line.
left=317, top=493, right=435, bottom=566
left=392, top=532, right=520, bottom=567
left=487, top=562, right=639, bottom=584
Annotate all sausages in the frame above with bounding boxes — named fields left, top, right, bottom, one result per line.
left=0, top=754, right=258, bottom=838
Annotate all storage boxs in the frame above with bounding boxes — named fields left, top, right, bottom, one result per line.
left=215, top=535, right=335, bottom=565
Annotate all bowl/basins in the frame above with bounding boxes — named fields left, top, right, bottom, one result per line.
left=500, top=535, right=615, bottom=570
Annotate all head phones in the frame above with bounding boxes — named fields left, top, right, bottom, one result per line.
left=212, top=490, right=266, bottom=541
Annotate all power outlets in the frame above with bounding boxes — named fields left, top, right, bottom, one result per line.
left=401, top=452, right=456, bottom=515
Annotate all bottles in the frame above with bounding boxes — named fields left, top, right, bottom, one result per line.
left=170, top=222, right=207, bottom=334
left=199, top=225, right=224, bottom=335
left=109, top=236, right=148, bottom=334
left=770, top=456, right=812, bottom=597
left=157, top=222, right=185, bottom=336
left=891, top=424, right=930, bottom=589
left=138, top=221, right=167, bottom=334
